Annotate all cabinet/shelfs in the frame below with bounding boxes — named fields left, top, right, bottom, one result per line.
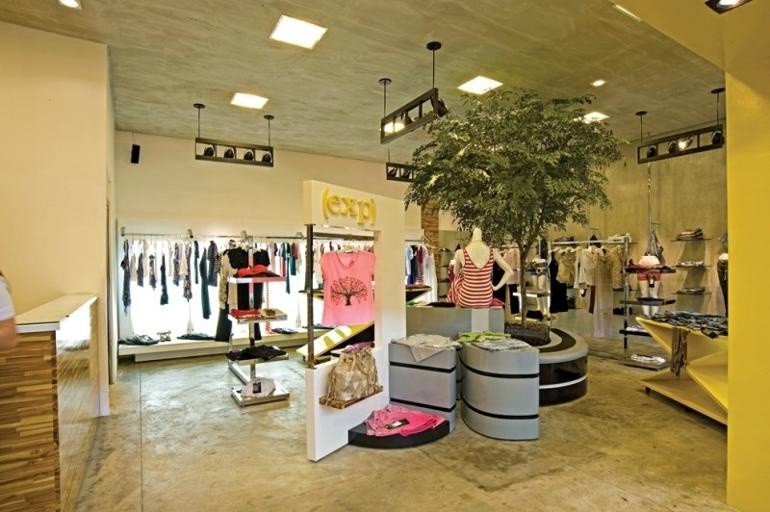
left=226, top=276, right=291, bottom=407
left=620, top=236, right=710, bottom=337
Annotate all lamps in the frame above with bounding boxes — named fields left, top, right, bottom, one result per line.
left=635, top=88, right=725, bottom=164
left=378, top=41, right=441, bottom=143
left=386, top=148, right=436, bottom=182
left=193, top=103, right=274, bottom=167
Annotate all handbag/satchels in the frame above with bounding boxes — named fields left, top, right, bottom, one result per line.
left=331, top=347, right=379, bottom=401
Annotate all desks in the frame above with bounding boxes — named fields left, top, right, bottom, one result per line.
left=389, top=335, right=458, bottom=433
left=457, top=332, right=541, bottom=441
left=633, top=316, right=728, bottom=427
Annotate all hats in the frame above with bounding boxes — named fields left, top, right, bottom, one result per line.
left=238, top=265, right=268, bottom=277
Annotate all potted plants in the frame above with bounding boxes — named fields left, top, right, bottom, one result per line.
left=398, top=89, right=634, bottom=343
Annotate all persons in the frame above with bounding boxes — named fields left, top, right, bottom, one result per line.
left=444, top=228, right=513, bottom=307
left=0, top=273, right=18, bottom=352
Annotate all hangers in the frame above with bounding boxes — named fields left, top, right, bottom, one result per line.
left=333, top=233, right=364, bottom=257
left=121, top=233, right=299, bottom=259
left=404, top=238, right=437, bottom=253
left=546, top=239, right=625, bottom=258
